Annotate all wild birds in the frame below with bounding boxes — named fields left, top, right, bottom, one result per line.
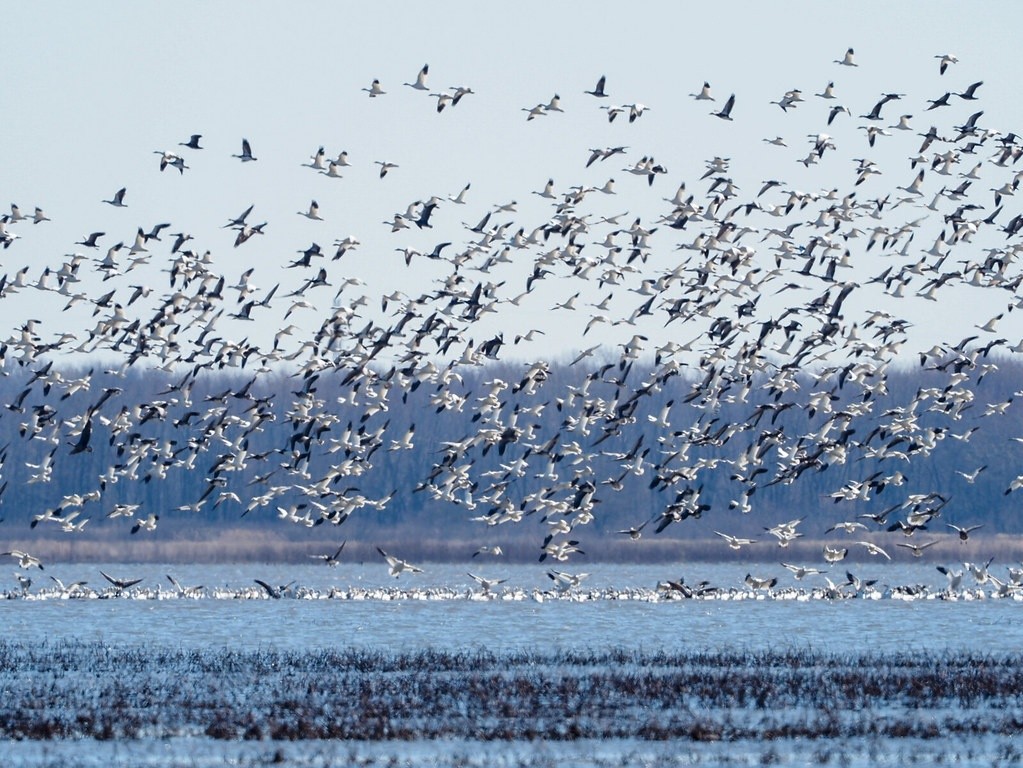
left=529, top=587, right=766, bottom=602
left=0, top=550, right=144, bottom=600
left=766, top=587, right=883, bottom=602
left=689, top=44, right=982, bottom=124
left=403, top=64, right=430, bottom=90
left=363, top=80, right=386, bottom=98
left=144, top=574, right=325, bottom=601
left=601, top=102, right=650, bottom=123
left=449, top=86, right=474, bottom=106
left=154, top=150, right=189, bottom=175
left=521, top=94, right=563, bottom=121
left=327, top=584, right=529, bottom=602
left=584, top=76, right=609, bottom=99
left=889, top=584, right=1023, bottom=602
left=428, top=93, right=453, bottom=113
left=231, top=138, right=257, bottom=163
left=179, top=134, right=204, bottom=149
left=1, top=111, right=1023, bottom=592
left=101, top=188, right=128, bottom=208
